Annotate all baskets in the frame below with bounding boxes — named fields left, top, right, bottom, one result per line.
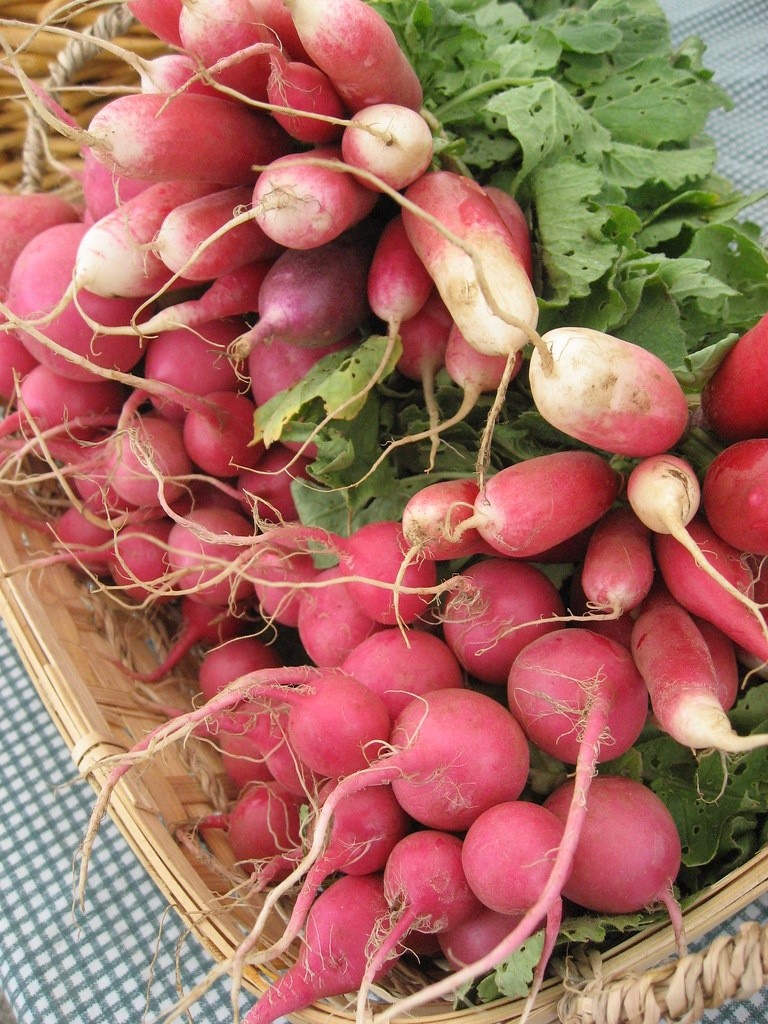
left=1, top=0, right=768, bottom=1024
